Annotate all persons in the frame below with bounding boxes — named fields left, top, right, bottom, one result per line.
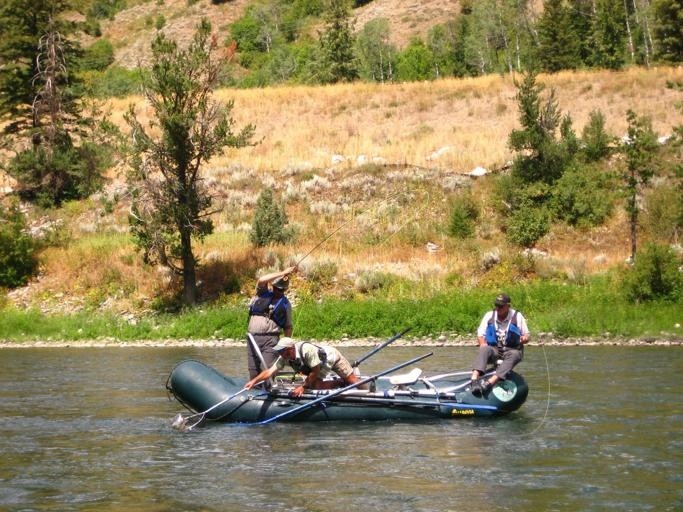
left=242, top=336, right=368, bottom=398
left=245, top=265, right=298, bottom=390
left=470, top=293, right=532, bottom=399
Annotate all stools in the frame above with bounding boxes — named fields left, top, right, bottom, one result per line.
left=387, top=367, right=423, bottom=396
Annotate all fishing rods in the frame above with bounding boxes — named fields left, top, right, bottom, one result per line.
left=295, top=188, right=430, bottom=264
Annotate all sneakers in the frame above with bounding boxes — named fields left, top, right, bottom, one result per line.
left=471, top=379, right=482, bottom=394
left=479, top=378, right=492, bottom=394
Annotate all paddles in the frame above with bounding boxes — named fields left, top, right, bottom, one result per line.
left=276, top=394, right=498, bottom=411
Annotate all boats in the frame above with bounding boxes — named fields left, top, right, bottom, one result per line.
left=165, top=355, right=531, bottom=425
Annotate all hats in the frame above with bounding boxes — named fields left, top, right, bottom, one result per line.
left=269, top=275, right=289, bottom=291
left=272, top=336, right=295, bottom=351
left=495, top=293, right=511, bottom=306
